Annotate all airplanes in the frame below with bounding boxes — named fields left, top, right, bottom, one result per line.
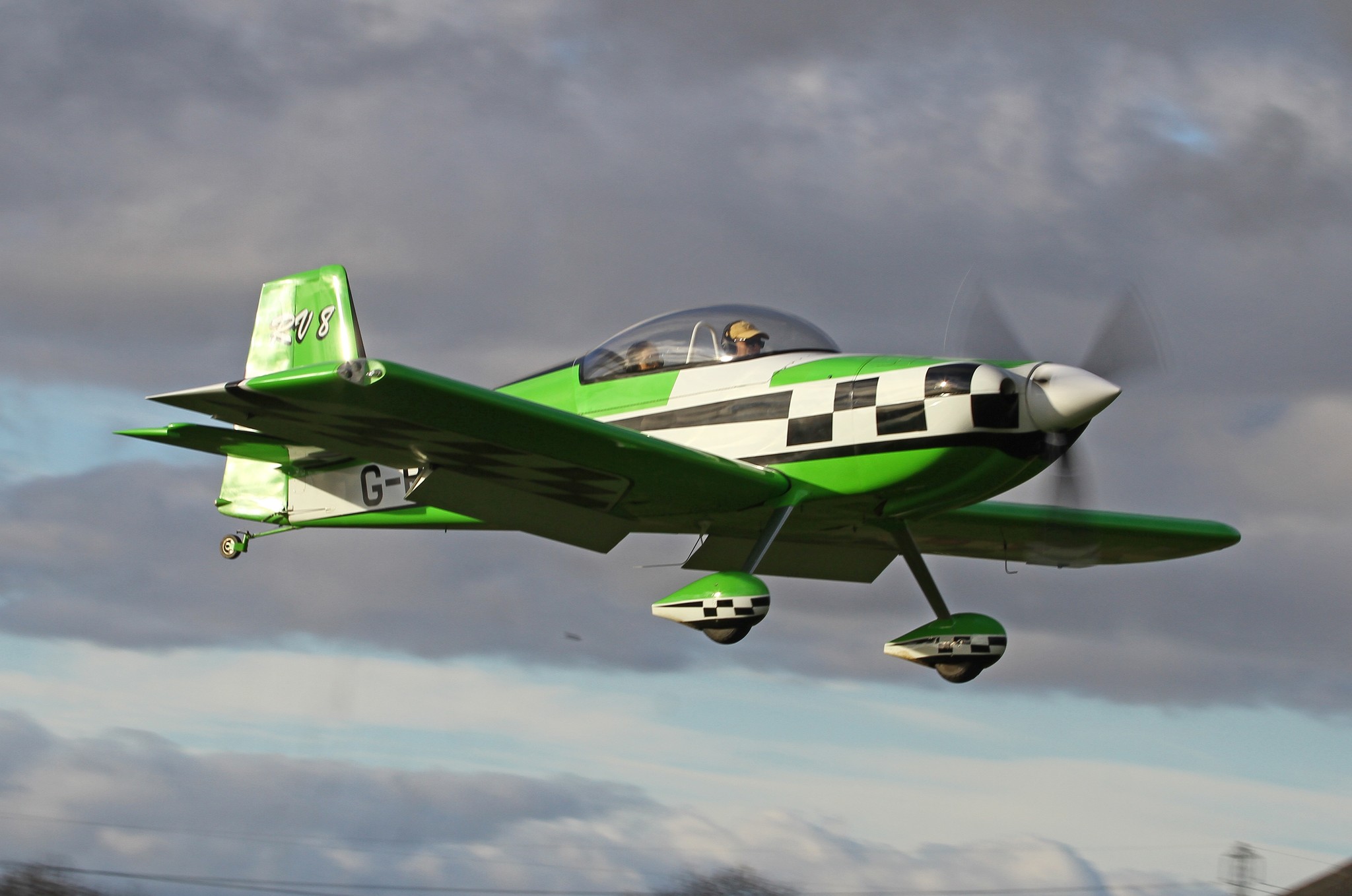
left=112, top=264, right=1241, bottom=684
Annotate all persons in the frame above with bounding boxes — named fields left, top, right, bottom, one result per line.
left=625, top=341, right=660, bottom=371
left=725, top=321, right=769, bottom=358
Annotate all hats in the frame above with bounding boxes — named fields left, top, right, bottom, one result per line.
left=724, top=321, right=769, bottom=340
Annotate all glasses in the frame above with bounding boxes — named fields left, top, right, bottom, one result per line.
left=733, top=338, right=765, bottom=348
left=636, top=356, right=665, bottom=366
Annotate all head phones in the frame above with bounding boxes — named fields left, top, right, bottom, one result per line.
left=721, top=321, right=737, bottom=355
left=623, top=341, right=664, bottom=373
